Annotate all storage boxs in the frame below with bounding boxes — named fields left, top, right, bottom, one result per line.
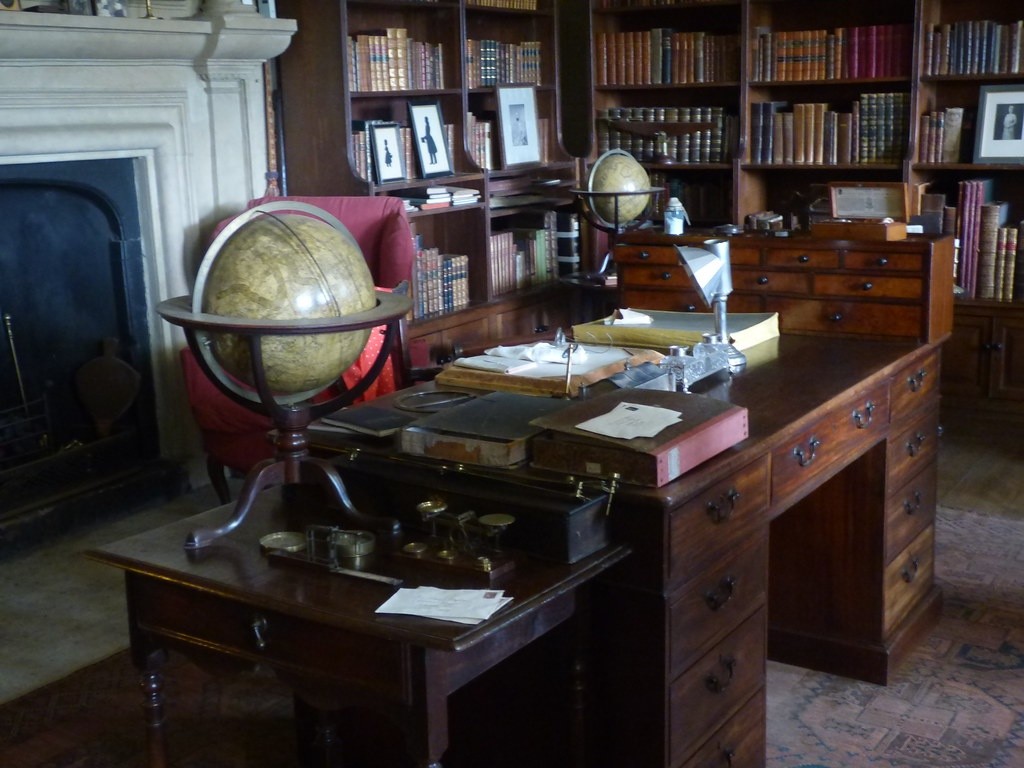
left=809, top=180, right=910, bottom=243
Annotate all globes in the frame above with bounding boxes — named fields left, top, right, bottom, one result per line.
left=567, top=147, right=666, bottom=278
left=153, top=200, right=414, bottom=550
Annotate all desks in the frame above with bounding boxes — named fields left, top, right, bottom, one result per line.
left=98, top=493, right=645, bottom=768
left=266, top=311, right=953, bottom=768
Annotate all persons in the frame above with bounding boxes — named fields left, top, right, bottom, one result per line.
left=1002, top=106, right=1017, bottom=139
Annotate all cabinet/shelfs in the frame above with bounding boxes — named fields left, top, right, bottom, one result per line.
left=277, top=0, right=1024, bottom=436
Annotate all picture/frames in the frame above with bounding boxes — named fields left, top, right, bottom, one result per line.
left=973, top=85, right=1024, bottom=164
left=495, top=82, right=546, bottom=170
left=406, top=100, right=457, bottom=179
left=369, top=124, right=407, bottom=186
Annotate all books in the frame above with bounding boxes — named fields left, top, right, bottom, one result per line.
left=306, top=406, right=418, bottom=436
left=911, top=20, right=1024, bottom=302
left=347, top=0, right=582, bottom=320
left=598, top=25, right=910, bottom=233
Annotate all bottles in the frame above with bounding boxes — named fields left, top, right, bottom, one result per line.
left=663, top=197, right=685, bottom=235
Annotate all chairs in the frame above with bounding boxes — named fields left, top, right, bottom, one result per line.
left=180, top=198, right=429, bottom=504
